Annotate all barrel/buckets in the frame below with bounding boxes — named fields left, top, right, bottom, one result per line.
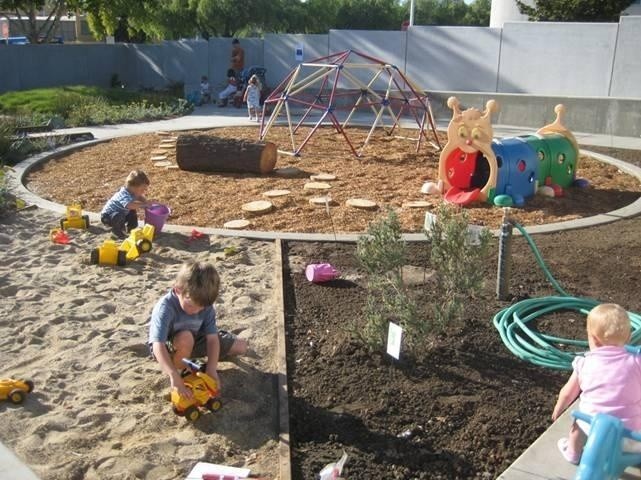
left=144, top=202, right=170, bottom=237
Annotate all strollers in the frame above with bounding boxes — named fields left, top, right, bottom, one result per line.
left=232, top=65, right=268, bottom=105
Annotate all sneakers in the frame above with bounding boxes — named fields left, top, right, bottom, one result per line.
left=557, top=438, right=580, bottom=464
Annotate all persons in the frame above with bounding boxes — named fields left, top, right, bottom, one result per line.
left=218, top=69, right=237, bottom=106
left=230, top=39, right=244, bottom=78
left=148, top=260, right=247, bottom=401
left=551, top=304, right=641, bottom=464
left=242, top=74, right=261, bottom=122
left=200, top=75, right=211, bottom=104
left=101, top=170, right=160, bottom=240
